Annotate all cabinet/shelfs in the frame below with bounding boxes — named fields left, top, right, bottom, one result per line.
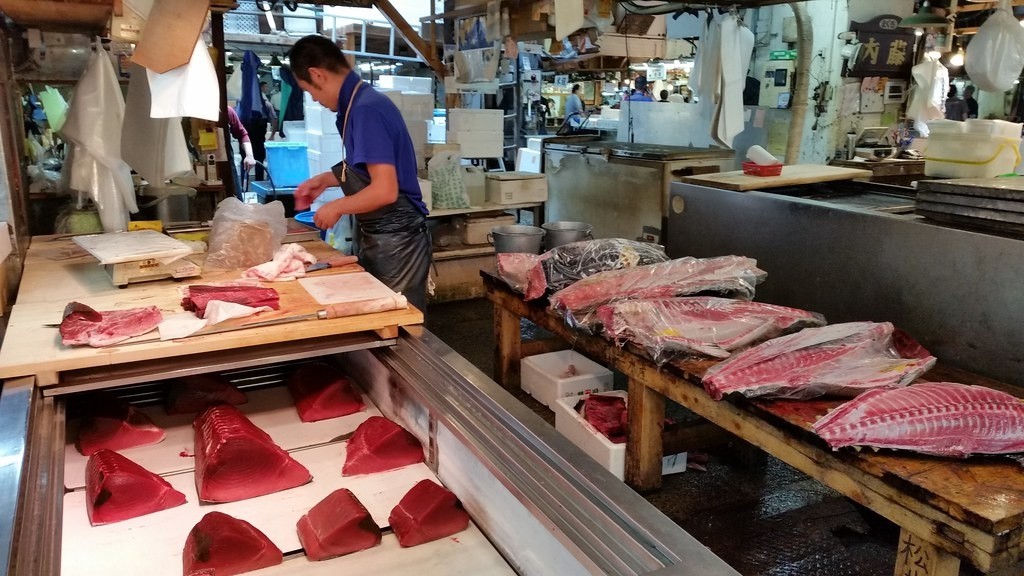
left=0, top=1, right=134, bottom=239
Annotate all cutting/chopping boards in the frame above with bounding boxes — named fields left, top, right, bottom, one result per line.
left=682, top=163, right=873, bottom=191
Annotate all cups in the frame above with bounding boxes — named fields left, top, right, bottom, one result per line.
left=745, top=145, right=779, bottom=165
left=846, top=131, right=857, bottom=159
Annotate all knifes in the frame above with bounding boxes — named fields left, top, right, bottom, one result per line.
left=305, top=256, right=359, bottom=271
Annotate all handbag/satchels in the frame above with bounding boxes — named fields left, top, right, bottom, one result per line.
left=204, top=198, right=288, bottom=266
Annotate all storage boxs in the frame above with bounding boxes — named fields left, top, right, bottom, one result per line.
left=601, top=31, right=668, bottom=59
left=556, top=391, right=688, bottom=480
left=264, top=72, right=550, bottom=244
left=923, top=132, right=1021, bottom=180
left=520, top=349, right=613, bottom=412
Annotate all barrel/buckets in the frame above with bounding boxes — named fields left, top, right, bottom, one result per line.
left=542, top=221, right=594, bottom=252
left=487, top=225, right=546, bottom=255
left=461, top=165, right=485, bottom=207
left=295, top=211, right=327, bottom=243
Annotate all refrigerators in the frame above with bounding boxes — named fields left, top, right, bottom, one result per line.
left=544, top=142, right=735, bottom=252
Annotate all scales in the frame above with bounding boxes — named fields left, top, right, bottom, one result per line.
left=105, top=255, right=202, bottom=289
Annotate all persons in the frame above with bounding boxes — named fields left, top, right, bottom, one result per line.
left=233, top=81, right=282, bottom=181
left=565, top=84, right=583, bottom=117
left=287, top=35, right=430, bottom=314
left=945, top=84, right=979, bottom=122
left=225, top=104, right=256, bottom=203
left=623, top=76, right=688, bottom=103
